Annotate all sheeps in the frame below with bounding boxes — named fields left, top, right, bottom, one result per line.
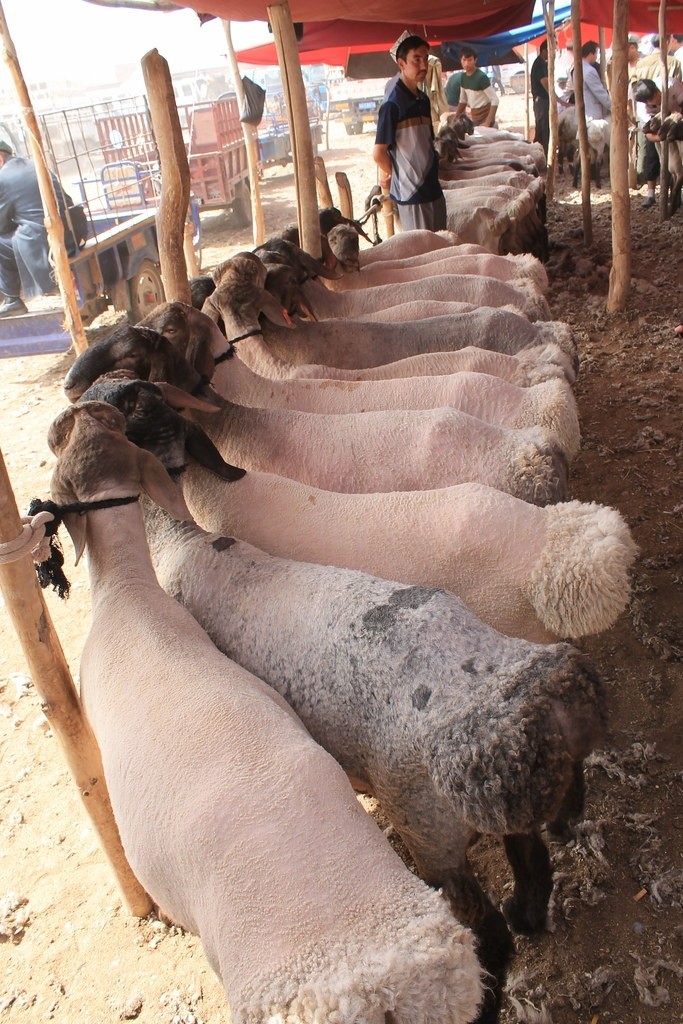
left=46, top=401, right=485, bottom=1024
left=130, top=280, right=581, bottom=452
left=557, top=105, right=610, bottom=189
left=80, top=402, right=607, bottom=985
left=365, top=114, right=548, bottom=258
left=46, top=371, right=637, bottom=659
left=640, top=111, right=683, bottom=224
left=63, top=321, right=568, bottom=511
left=193, top=208, right=576, bottom=370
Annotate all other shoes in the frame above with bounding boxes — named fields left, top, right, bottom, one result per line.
left=641, top=197, right=656, bottom=208
left=0, top=299, right=28, bottom=318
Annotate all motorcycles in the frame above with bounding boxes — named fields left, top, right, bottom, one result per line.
left=1, top=160, right=204, bottom=359
left=256, top=79, right=328, bottom=171
left=95, top=90, right=268, bottom=228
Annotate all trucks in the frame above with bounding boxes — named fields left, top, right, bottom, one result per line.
left=324, top=60, right=394, bottom=136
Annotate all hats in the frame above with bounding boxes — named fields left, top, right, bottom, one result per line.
left=628, top=34, right=640, bottom=43
left=0, top=141, right=12, bottom=155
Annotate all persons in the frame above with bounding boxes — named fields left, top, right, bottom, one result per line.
left=0, top=140, right=87, bottom=318
left=530, top=33, right=683, bottom=208
left=443, top=46, right=499, bottom=127
left=373, top=30, right=446, bottom=231
